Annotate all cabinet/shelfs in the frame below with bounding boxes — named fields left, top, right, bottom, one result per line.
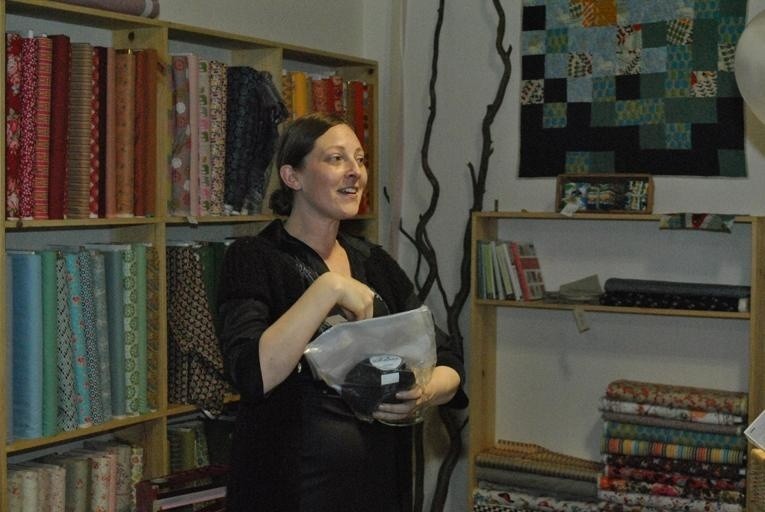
left=467, top=211, right=762, bottom=512
left=2, top=1, right=387, bottom=458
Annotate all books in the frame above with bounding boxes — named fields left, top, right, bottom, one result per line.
left=476, top=238, right=546, bottom=302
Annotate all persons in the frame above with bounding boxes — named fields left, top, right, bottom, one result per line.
left=217, top=111, right=468, bottom=512
